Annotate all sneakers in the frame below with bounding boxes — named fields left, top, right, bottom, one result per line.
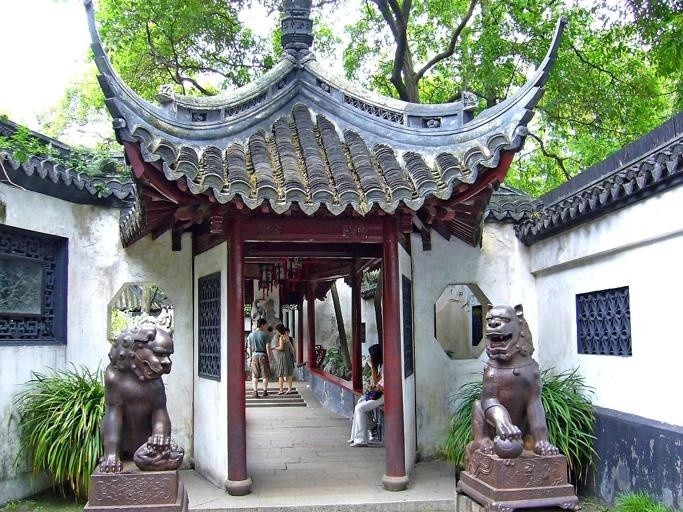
left=253, top=391, right=258, bottom=397
left=346, top=439, right=369, bottom=447
left=262, top=389, right=268, bottom=396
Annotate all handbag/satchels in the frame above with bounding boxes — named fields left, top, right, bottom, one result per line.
left=364, top=389, right=383, bottom=401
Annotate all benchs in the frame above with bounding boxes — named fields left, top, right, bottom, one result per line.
left=309, top=366, right=385, bottom=440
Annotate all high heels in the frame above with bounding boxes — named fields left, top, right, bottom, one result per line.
left=277, top=390, right=292, bottom=395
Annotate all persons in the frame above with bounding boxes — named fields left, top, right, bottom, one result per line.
left=245, top=318, right=295, bottom=398
left=344, top=342, right=383, bottom=448
